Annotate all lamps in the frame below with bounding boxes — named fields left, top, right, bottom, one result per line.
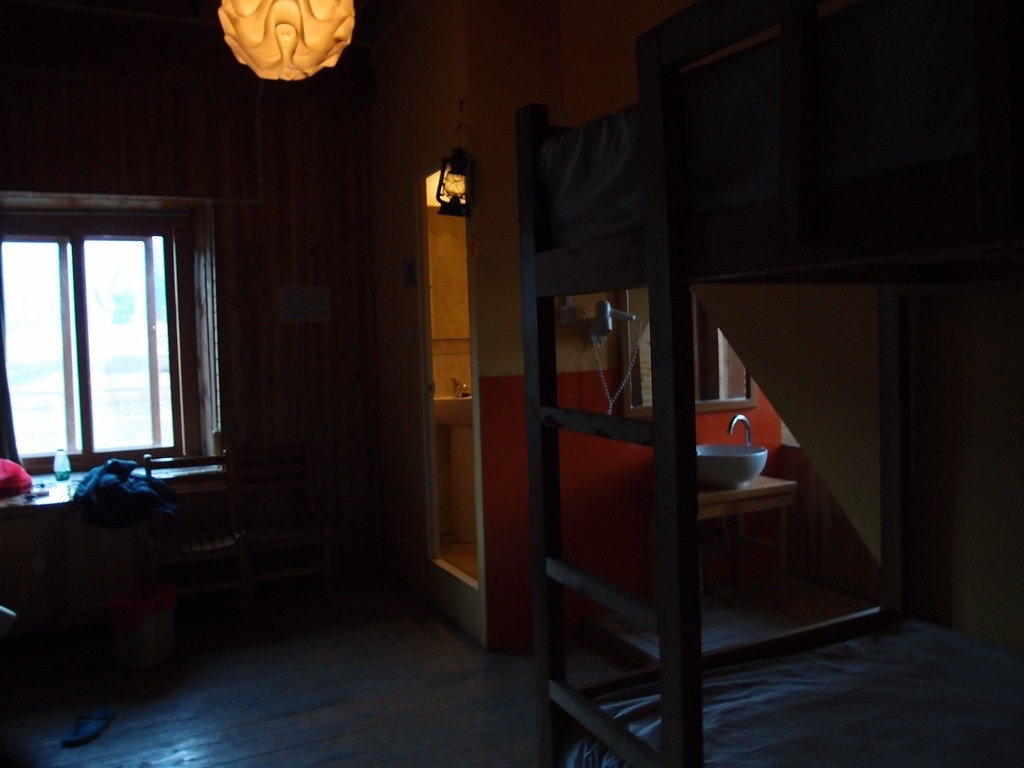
left=217, top=0, right=357, bottom=83
left=436, top=145, right=478, bottom=218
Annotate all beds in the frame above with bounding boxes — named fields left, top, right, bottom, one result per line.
left=523, top=603, right=1024, bottom=768
left=511, top=0, right=1024, bottom=298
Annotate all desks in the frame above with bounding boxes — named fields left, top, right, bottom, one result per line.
left=639, top=474, right=799, bottom=613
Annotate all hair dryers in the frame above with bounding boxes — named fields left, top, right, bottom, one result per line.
left=594, top=300, right=636, bottom=334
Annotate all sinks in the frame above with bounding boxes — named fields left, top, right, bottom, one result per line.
left=435, top=393, right=475, bottom=426
left=695, top=443, right=769, bottom=492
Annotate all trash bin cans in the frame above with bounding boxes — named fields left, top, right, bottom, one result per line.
left=107, top=588, right=176, bottom=669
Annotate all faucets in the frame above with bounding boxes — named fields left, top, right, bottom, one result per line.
left=726, top=413, right=753, bottom=447
left=449, top=376, right=468, bottom=398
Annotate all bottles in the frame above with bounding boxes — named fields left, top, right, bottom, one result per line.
left=53, top=448, right=72, bottom=500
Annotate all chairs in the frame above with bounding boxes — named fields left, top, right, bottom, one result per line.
left=223, top=438, right=335, bottom=616
left=140, top=447, right=252, bottom=624
